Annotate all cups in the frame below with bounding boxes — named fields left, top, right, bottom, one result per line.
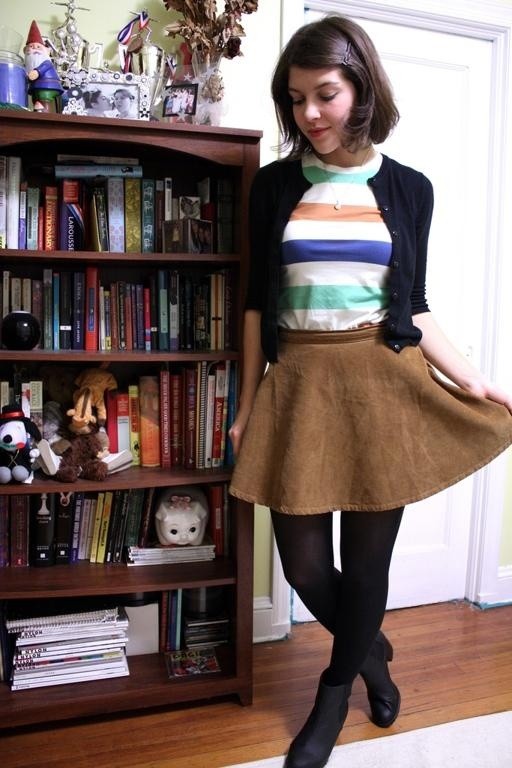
left=0, top=51, right=29, bottom=108
left=1, top=23, right=24, bottom=55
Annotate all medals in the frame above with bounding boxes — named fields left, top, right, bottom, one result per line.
left=127, top=32, right=143, bottom=52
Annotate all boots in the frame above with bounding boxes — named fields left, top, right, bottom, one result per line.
left=282, top=665, right=353, bottom=768
left=358, top=632, right=400, bottom=728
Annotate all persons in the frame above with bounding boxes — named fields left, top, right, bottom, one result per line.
left=226, top=15, right=512, bottom=765
left=84, top=89, right=135, bottom=117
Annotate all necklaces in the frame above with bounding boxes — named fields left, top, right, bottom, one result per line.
left=320, top=148, right=372, bottom=210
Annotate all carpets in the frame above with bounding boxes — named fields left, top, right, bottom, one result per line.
left=225, top=708, right=512, bottom=767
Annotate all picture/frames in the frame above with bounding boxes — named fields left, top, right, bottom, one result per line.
left=63, top=70, right=149, bottom=119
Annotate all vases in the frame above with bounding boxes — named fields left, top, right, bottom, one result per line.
left=190, top=50, right=223, bottom=126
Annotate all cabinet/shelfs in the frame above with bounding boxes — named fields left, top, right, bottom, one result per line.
left=0, top=106, right=251, bottom=733
